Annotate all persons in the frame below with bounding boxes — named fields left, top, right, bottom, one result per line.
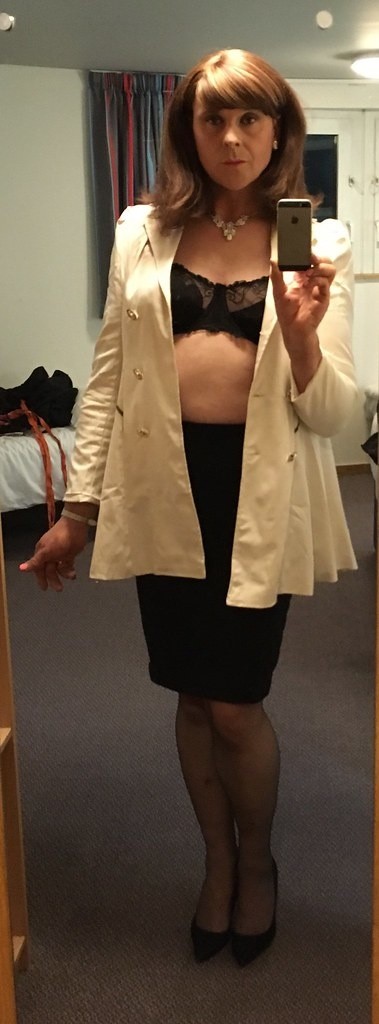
left=20, top=49, right=359, bottom=969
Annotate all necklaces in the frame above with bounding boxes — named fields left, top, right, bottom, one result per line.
left=208, top=210, right=267, bottom=242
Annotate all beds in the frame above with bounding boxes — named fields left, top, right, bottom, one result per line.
left=0, top=413, right=75, bottom=514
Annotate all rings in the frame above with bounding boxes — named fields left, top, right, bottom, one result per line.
left=56, top=560, right=66, bottom=565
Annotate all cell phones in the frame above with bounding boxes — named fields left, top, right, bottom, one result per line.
left=276, top=199, right=312, bottom=272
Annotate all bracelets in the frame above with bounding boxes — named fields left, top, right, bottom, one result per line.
left=61, top=509, right=97, bottom=527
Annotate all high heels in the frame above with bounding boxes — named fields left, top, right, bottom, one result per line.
left=230, top=855, right=279, bottom=967
left=191, top=915, right=231, bottom=964
